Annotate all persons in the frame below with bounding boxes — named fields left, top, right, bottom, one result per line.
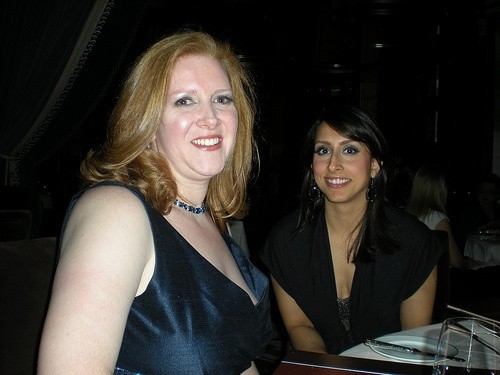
left=406, top=164, right=462, bottom=268
left=36, top=35, right=287, bottom=375
left=258, top=109, right=440, bottom=354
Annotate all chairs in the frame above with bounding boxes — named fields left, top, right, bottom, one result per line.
left=464, top=231, right=499, bottom=265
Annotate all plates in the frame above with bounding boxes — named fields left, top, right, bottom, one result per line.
left=371, top=334, right=459, bottom=366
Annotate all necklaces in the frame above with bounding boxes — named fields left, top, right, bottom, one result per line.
left=172, top=199, right=206, bottom=214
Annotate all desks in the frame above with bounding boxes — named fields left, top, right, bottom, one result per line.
left=339, top=322, right=499, bottom=370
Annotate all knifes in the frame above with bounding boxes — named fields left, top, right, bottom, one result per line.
left=362, top=338, right=467, bottom=364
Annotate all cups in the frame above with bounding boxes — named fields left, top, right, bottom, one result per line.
left=433, top=316, right=500, bottom=375
